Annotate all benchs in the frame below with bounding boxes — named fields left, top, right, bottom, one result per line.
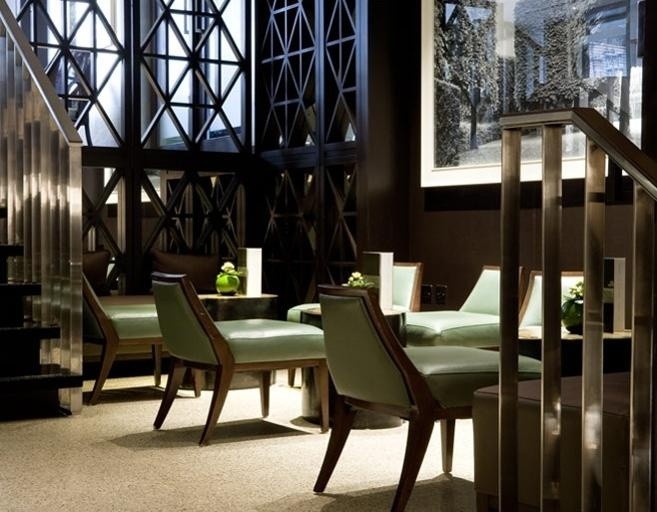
left=474, top=373, right=657, bottom=512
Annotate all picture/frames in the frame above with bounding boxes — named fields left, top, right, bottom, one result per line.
left=421, top=0, right=644, bottom=189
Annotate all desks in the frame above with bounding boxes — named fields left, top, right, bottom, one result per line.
left=519, top=329, right=634, bottom=371
